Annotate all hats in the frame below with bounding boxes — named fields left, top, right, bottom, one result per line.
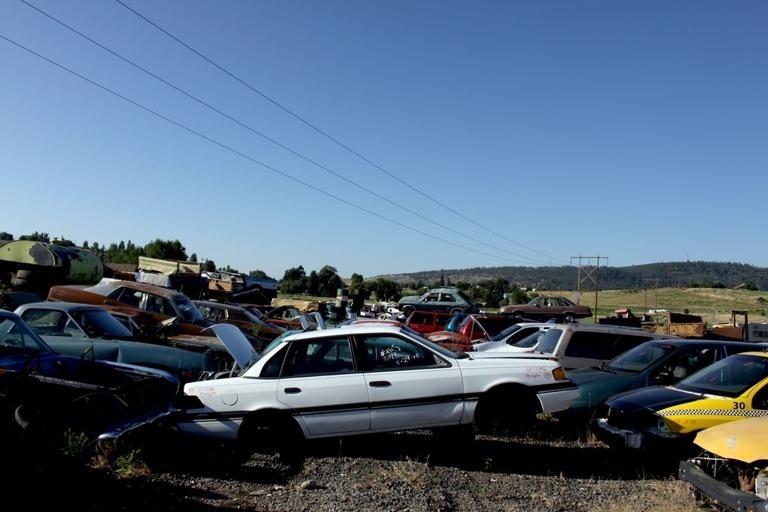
left=354, top=283, right=524, bottom=348
left=181, top=324, right=586, bottom=460
left=499, top=294, right=594, bottom=323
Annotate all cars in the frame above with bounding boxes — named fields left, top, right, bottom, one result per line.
left=564, top=337, right=768, bottom=438
left=181, top=324, right=586, bottom=460
left=44, top=277, right=263, bottom=363
left=0, top=238, right=330, bottom=357
left=499, top=294, right=594, bottom=323
left=178, top=299, right=290, bottom=345
left=0, top=299, right=219, bottom=383
left=0, top=309, right=180, bottom=459
left=586, top=351, right=768, bottom=472
left=676, top=415, right=768, bottom=511
left=354, top=283, right=524, bottom=348
left=489, top=318, right=694, bottom=371
